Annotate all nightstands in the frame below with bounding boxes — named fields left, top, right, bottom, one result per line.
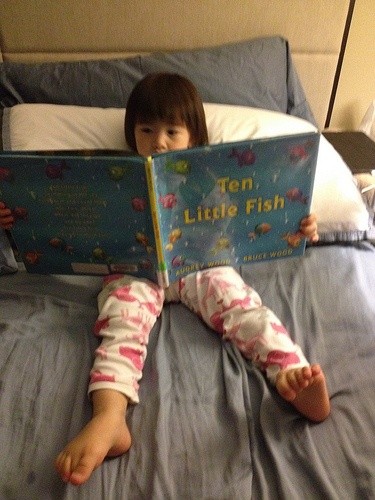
left=321, top=131, right=375, bottom=212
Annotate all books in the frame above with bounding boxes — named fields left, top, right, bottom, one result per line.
left=0, top=130, right=320, bottom=289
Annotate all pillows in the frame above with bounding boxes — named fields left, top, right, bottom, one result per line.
left=2, top=103, right=375, bottom=272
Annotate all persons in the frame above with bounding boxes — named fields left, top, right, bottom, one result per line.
left=0, top=71, right=331, bottom=486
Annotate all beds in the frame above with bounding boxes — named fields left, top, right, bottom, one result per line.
left=0, top=34, right=375, bottom=500
